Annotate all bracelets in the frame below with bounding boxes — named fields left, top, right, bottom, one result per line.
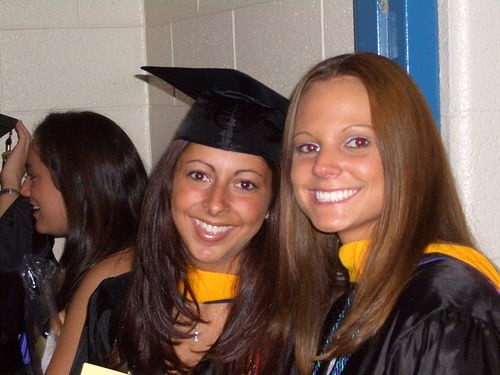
left=1, top=189, right=20, bottom=197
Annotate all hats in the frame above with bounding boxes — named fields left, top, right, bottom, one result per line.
left=141, top=66, right=291, bottom=163
left=0, top=113, right=19, bottom=170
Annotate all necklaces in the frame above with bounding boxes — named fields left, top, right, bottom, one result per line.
left=313, top=287, right=363, bottom=375
left=183, top=301, right=229, bottom=343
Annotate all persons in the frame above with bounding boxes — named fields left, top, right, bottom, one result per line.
left=262, top=51, right=500, bottom=375
left=69, top=67, right=291, bottom=375
left=0, top=113, right=60, bottom=375
left=21, top=110, right=149, bottom=375
